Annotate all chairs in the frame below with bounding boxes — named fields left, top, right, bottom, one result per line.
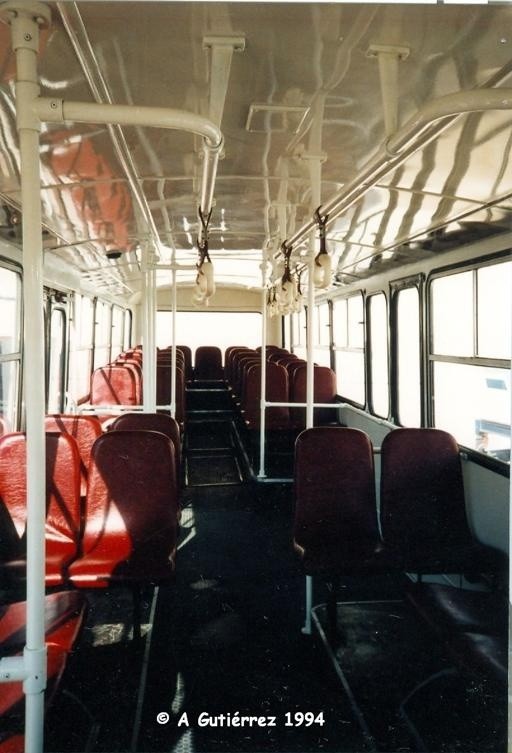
left=193, top=345, right=223, bottom=379
left=39, top=126, right=139, bottom=253
